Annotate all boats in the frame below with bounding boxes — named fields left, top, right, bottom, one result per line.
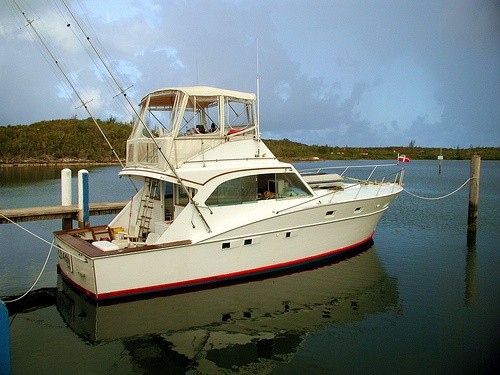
left=1, top=1, right=404, bottom=303
left=54, top=236, right=404, bottom=375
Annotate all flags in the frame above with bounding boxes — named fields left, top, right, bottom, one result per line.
left=398, top=152, right=410, bottom=162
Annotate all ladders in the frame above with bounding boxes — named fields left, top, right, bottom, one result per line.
left=134, top=177, right=158, bottom=243
